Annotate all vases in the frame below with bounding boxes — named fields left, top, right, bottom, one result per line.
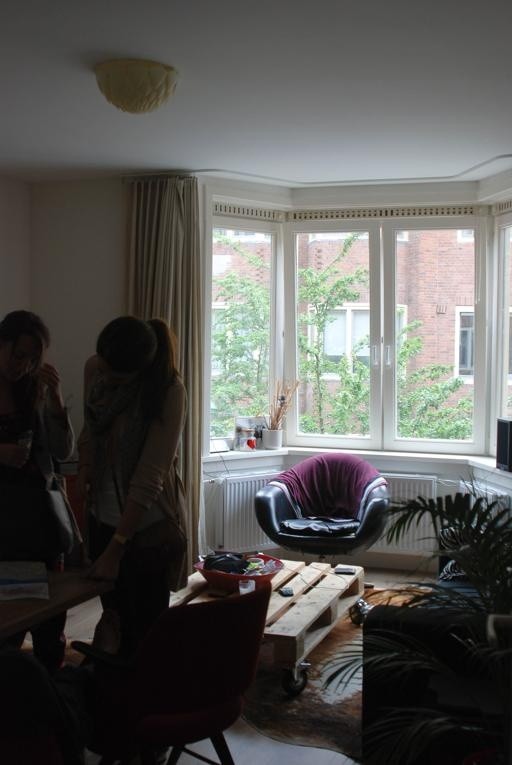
left=262, top=430, right=282, bottom=449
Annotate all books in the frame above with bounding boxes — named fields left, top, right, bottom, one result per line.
left=1, top=561, right=50, bottom=601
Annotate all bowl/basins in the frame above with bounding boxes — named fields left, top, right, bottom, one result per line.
left=193, top=555, right=282, bottom=587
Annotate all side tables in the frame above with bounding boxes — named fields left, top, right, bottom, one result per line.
left=0, top=571, right=113, bottom=639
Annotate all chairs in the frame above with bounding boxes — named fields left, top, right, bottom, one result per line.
left=0, top=645, right=97, bottom=765
left=254, top=453, right=388, bottom=563
left=361, top=528, right=512, bottom=765
left=71, top=584, right=269, bottom=765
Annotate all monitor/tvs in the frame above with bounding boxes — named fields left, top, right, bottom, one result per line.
left=496, top=418, right=512, bottom=471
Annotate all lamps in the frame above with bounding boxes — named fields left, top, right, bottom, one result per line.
left=93, top=59, right=178, bottom=114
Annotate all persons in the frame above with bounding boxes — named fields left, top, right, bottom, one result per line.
left=2, top=308, right=82, bottom=661
left=73, top=316, right=192, bottom=641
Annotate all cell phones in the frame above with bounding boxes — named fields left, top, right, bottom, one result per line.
left=279, top=587, right=293, bottom=597
left=335, top=568, right=355, bottom=574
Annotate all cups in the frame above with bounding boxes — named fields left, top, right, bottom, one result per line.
left=237, top=578, right=257, bottom=596
left=261, top=429, right=283, bottom=451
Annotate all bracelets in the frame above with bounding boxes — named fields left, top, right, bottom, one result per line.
left=111, top=531, right=131, bottom=548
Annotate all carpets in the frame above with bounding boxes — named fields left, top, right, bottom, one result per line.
left=241, top=588, right=434, bottom=763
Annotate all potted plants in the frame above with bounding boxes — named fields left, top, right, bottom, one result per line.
left=327, top=494, right=512, bottom=765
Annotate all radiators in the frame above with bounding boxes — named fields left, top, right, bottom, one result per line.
left=219, top=473, right=437, bottom=557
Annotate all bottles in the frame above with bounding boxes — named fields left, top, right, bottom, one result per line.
left=234, top=429, right=257, bottom=452
